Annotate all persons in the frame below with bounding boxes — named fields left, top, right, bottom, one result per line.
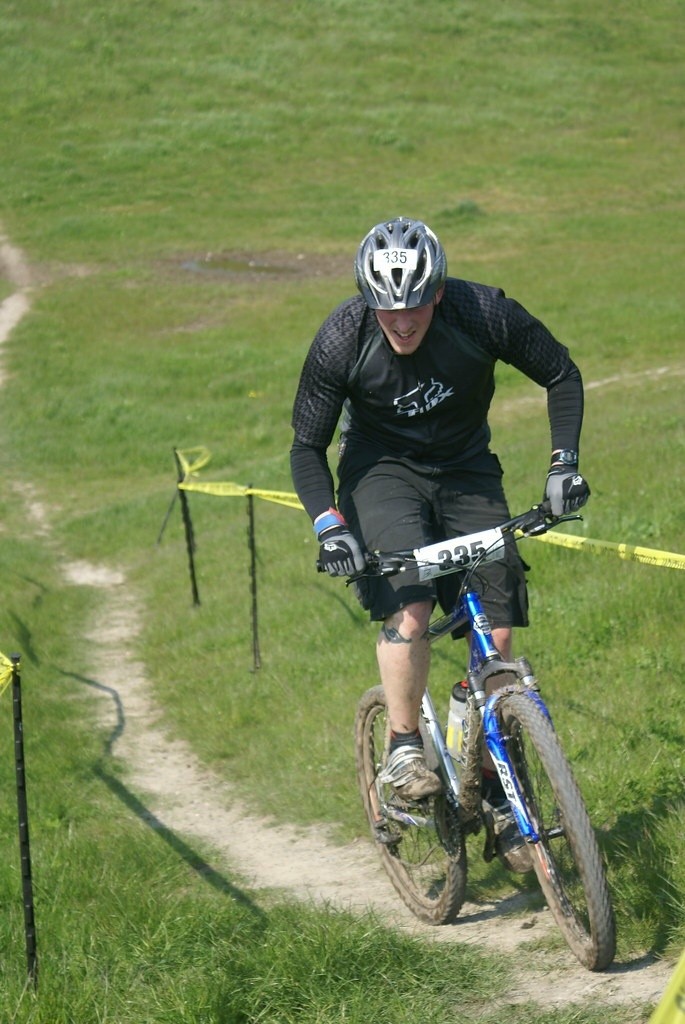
left=287, top=215, right=586, bottom=876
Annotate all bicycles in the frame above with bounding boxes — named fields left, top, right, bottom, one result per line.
left=313, top=498, right=617, bottom=974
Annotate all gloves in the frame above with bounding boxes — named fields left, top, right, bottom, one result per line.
left=319, top=525, right=366, bottom=577
left=543, top=464, right=590, bottom=517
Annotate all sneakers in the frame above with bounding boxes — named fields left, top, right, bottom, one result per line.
left=378, top=745, right=441, bottom=802
left=482, top=785, right=532, bottom=872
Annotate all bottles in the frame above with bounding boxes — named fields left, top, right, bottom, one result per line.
left=446, top=681, right=469, bottom=761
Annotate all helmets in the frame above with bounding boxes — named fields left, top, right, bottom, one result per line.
left=354, top=216, right=447, bottom=310
